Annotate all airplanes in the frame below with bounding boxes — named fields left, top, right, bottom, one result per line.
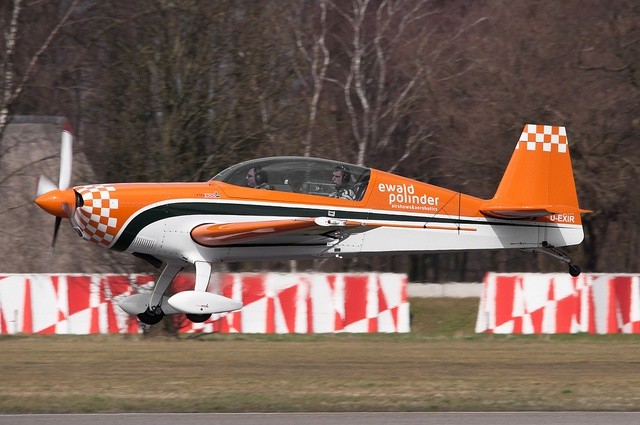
left=35, top=124, right=594, bottom=324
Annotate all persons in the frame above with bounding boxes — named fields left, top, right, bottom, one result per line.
left=328, top=166, right=357, bottom=202
left=245, top=167, right=271, bottom=191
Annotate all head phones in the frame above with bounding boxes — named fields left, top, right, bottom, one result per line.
left=254, top=167, right=262, bottom=184
left=336, top=166, right=348, bottom=182
